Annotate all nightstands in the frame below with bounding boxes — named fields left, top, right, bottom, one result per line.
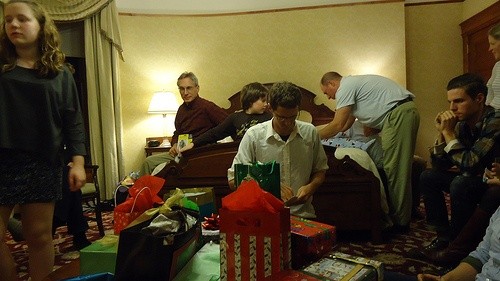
left=144, top=136, right=174, bottom=158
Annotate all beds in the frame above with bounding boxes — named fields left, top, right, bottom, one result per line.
left=154, top=82, right=388, bottom=243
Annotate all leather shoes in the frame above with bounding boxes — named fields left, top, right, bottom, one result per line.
left=415, top=236, right=449, bottom=259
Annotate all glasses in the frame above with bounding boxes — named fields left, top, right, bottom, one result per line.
left=271, top=107, right=300, bottom=121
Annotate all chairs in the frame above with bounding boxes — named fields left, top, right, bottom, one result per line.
left=79, top=165, right=105, bottom=236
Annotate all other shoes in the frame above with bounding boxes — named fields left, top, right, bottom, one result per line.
left=72, top=238, right=92, bottom=251
left=393, top=223, right=410, bottom=233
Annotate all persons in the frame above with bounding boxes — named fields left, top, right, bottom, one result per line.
left=169, top=82, right=273, bottom=158
left=141, top=72, right=237, bottom=177
left=227, top=81, right=330, bottom=222
left=317, top=72, right=420, bottom=234
left=0, top=0, right=86, bottom=281
left=485, top=25, right=500, bottom=113
left=417, top=73, right=500, bottom=281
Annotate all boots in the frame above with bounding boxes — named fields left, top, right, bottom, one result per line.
left=426, top=206, right=493, bottom=267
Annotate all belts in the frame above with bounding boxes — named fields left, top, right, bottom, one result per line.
left=390, top=96, right=414, bottom=111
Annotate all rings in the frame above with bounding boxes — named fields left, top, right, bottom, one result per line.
left=482, top=176, right=489, bottom=184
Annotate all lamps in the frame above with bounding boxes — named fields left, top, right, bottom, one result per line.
left=147, top=91, right=180, bottom=118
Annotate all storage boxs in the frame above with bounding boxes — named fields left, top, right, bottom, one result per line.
left=78, top=234, right=119, bottom=274
left=302, top=249, right=385, bottom=281
left=288, top=215, right=336, bottom=269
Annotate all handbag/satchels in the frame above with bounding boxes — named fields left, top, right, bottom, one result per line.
left=113, top=205, right=203, bottom=281
left=233, top=158, right=281, bottom=201
left=113, top=184, right=155, bottom=236
